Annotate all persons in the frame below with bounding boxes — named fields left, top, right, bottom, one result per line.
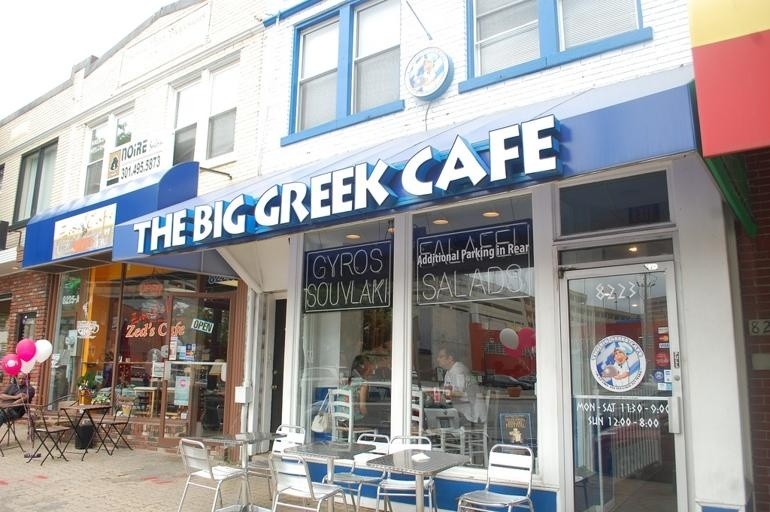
left=611, top=347, right=630, bottom=387
left=436, top=346, right=487, bottom=454
left=0, top=371, right=35, bottom=429
left=368, top=331, right=392, bottom=398
left=422, top=51, right=438, bottom=83
left=336, top=355, right=386, bottom=427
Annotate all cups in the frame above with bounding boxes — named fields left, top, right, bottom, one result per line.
left=76, top=321, right=99, bottom=336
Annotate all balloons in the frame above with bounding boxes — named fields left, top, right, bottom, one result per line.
left=35, top=339, right=53, bottom=363
left=499, top=326, right=536, bottom=360
left=20, top=355, right=36, bottom=374
left=16, top=338, right=36, bottom=362
left=1, top=353, right=21, bottom=378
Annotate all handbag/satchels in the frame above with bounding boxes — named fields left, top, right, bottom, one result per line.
left=311, top=390, right=334, bottom=433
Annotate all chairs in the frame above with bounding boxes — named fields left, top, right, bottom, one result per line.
left=179, top=438, right=250, bottom=512
left=25, top=403, right=71, bottom=466
left=326, top=388, right=379, bottom=444
left=94, top=403, right=133, bottom=455
left=248, top=424, right=306, bottom=500
left=457, top=443, right=535, bottom=512
left=321, top=433, right=393, bottom=512
left=375, top=435, right=438, bottom=512
left=411, top=390, right=444, bottom=451
left=268, top=454, right=348, bottom=511
left=456, top=389, right=490, bottom=469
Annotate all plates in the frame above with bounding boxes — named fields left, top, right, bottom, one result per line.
left=77, top=337, right=96, bottom=339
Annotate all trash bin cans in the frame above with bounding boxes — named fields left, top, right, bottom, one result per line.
left=74, top=423, right=95, bottom=449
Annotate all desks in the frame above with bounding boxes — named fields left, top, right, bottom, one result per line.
left=202, top=430, right=288, bottom=505
left=56, top=405, right=113, bottom=461
left=383, top=408, right=460, bottom=430
left=0, top=402, right=29, bottom=452
left=284, top=440, right=376, bottom=511
left=366, top=449, right=470, bottom=511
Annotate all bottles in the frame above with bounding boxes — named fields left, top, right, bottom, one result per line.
left=434, top=387, right=441, bottom=403
left=444, top=381, right=453, bottom=403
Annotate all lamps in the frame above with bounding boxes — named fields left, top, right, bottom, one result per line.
left=208, top=359, right=226, bottom=392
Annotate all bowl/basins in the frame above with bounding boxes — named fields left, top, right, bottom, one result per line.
left=508, top=387, right=522, bottom=397
